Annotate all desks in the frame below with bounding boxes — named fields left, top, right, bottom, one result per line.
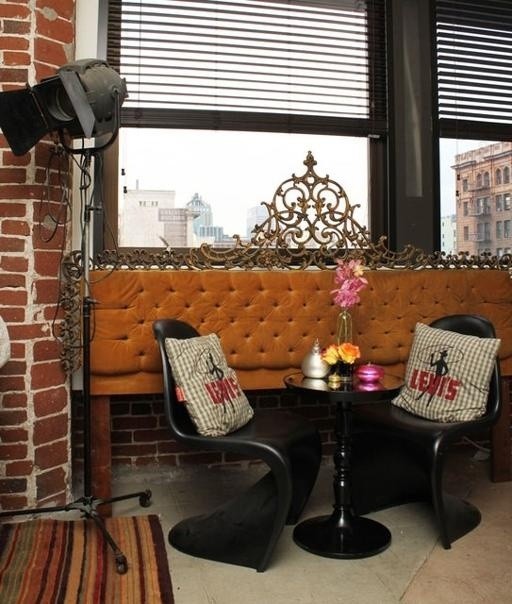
left=282, top=372, right=404, bottom=560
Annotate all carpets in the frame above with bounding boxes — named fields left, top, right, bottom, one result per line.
left=1, top=515, right=176, bottom=604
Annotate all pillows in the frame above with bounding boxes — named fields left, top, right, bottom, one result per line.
left=392, top=322, right=502, bottom=422
left=164, top=333, right=255, bottom=438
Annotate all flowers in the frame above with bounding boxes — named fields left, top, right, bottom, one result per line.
left=325, top=342, right=359, bottom=365
left=326, top=255, right=371, bottom=311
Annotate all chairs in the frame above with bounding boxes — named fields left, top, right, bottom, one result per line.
left=355, top=312, right=501, bottom=550
left=152, top=318, right=323, bottom=573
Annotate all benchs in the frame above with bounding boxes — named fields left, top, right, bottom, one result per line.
left=56, top=145, right=512, bottom=510
